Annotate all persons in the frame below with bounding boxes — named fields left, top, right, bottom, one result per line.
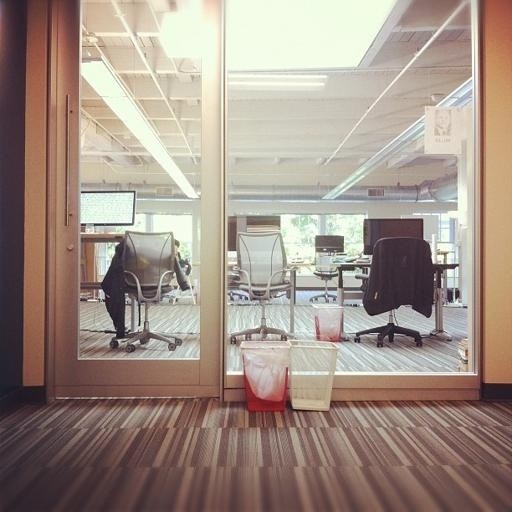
left=433, top=110, right=451, bottom=137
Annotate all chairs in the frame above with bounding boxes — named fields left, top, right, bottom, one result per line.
left=310, top=235, right=346, bottom=304
left=353, top=236, right=435, bottom=349
left=228, top=229, right=298, bottom=346
left=107, top=230, right=186, bottom=352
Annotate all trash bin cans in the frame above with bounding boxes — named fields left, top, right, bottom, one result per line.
left=240, top=340, right=290, bottom=412
left=288, top=339, right=340, bottom=413
left=311, top=303, right=344, bottom=342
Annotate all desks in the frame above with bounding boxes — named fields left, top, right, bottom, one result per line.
left=333, top=256, right=461, bottom=343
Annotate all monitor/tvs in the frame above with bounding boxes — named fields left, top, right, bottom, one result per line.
left=363, top=217, right=424, bottom=255
left=80, top=191, right=136, bottom=233
left=228, top=215, right=281, bottom=251
left=314, top=235, right=344, bottom=256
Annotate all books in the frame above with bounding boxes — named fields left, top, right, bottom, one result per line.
left=356, top=258, right=370, bottom=263
